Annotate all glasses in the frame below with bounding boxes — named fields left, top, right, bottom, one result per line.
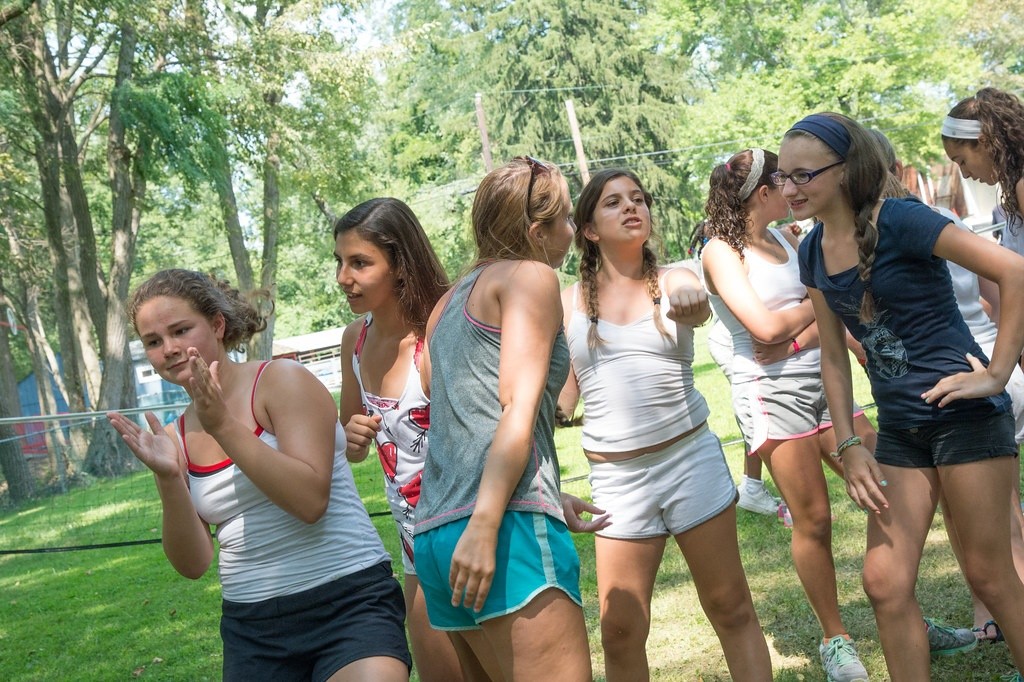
left=513, top=155, right=550, bottom=221
left=769, top=158, right=848, bottom=186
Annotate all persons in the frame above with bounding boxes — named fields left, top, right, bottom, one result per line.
left=868, top=129, right=1024, bottom=645
left=419, top=152, right=613, bottom=682
left=775, top=113, right=1024, bottom=682
left=330, top=197, right=488, bottom=682
left=687, top=212, right=785, bottom=518
left=551, top=167, right=777, bottom=682
left=104, top=269, right=414, bottom=681
left=941, top=87, right=1024, bottom=374
left=698, top=146, right=977, bottom=682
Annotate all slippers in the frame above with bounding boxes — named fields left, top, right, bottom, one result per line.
left=971, top=620, right=1005, bottom=645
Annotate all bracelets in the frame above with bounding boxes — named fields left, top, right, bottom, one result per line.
left=790, top=336, right=800, bottom=355
left=829, top=434, right=862, bottom=463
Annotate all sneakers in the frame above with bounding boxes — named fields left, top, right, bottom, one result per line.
left=818, top=635, right=870, bottom=682
left=922, top=617, right=980, bottom=656
left=737, top=486, right=780, bottom=516
